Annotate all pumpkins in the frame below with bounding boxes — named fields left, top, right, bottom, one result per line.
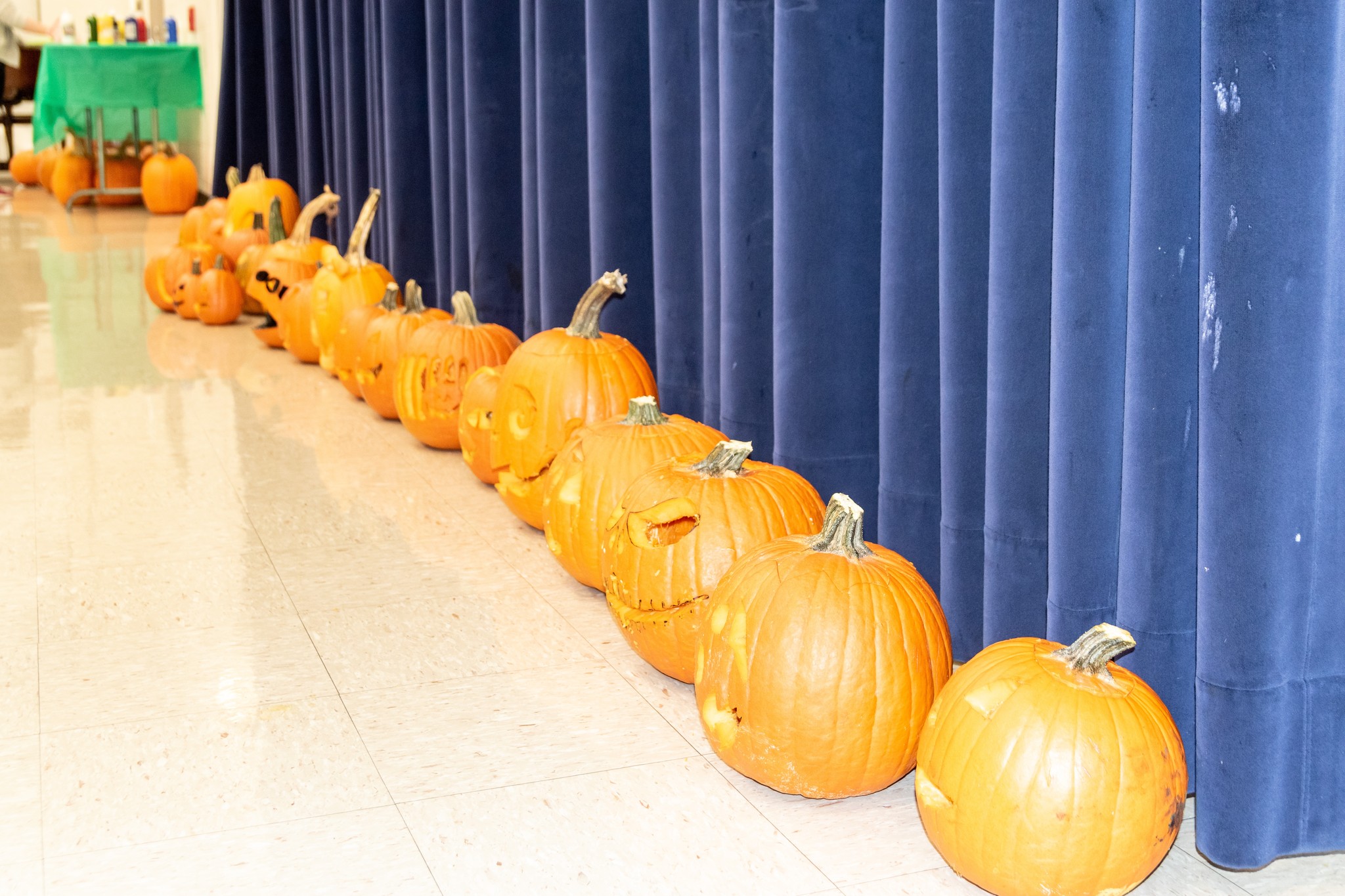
left=5, top=127, right=197, bottom=215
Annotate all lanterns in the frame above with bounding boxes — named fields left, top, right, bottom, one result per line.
left=243, top=185, right=340, bottom=350
left=459, top=364, right=508, bottom=483
left=333, top=281, right=406, bottom=398
left=490, top=268, right=661, bottom=532
left=354, top=279, right=455, bottom=418
left=604, top=439, right=827, bottom=686
left=310, top=187, right=402, bottom=377
left=174, top=254, right=204, bottom=319
left=392, top=290, right=522, bottom=448
left=916, top=623, right=1189, bottom=896
left=693, top=492, right=952, bottom=801
left=541, top=393, right=732, bottom=595
left=145, top=253, right=176, bottom=311
left=181, top=163, right=299, bottom=312
left=194, top=253, right=244, bottom=326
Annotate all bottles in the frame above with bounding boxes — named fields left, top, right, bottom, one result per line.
left=166, top=13, right=178, bottom=45
left=85, top=12, right=146, bottom=44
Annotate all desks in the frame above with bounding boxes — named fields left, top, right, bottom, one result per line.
left=33, top=44, right=204, bottom=213
